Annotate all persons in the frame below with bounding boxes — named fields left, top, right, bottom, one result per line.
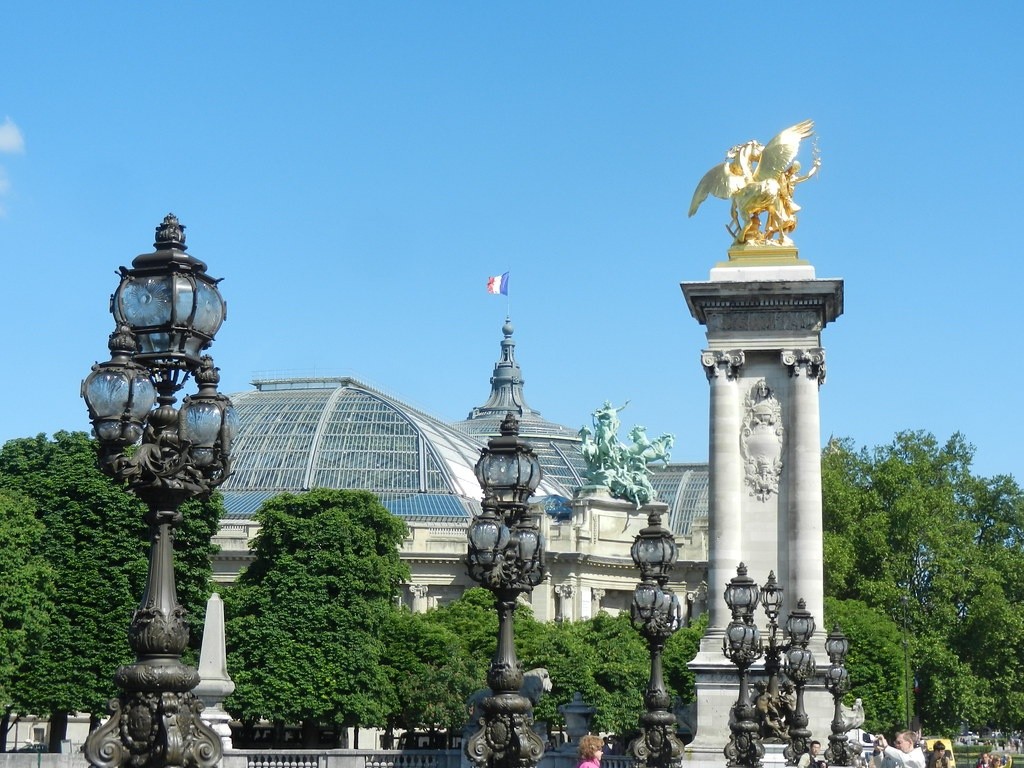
left=576, top=735, right=610, bottom=768
left=765, top=159, right=821, bottom=245
left=740, top=379, right=788, bottom=503
left=577, top=400, right=657, bottom=503
left=797, top=729, right=1024, bottom=768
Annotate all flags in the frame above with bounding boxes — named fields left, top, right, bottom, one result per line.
left=488, top=271, right=510, bottom=296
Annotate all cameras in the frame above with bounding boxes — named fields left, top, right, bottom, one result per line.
left=874, top=738, right=882, bottom=747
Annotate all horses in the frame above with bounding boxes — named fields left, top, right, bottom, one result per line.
left=687, top=118, right=815, bottom=246
left=577, top=419, right=676, bottom=478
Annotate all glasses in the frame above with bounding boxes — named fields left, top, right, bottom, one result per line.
left=893, top=740, right=908, bottom=744
